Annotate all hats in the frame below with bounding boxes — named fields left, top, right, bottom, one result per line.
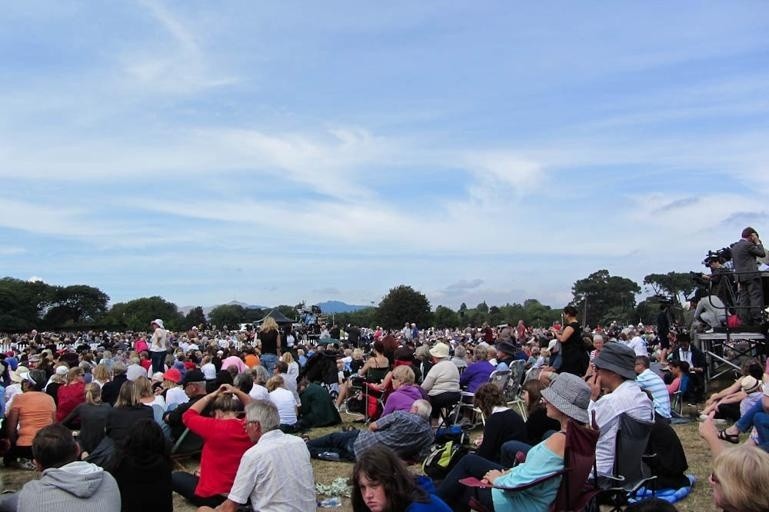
left=176, top=369, right=207, bottom=386
left=7, top=365, right=37, bottom=385
left=496, top=340, right=516, bottom=355
left=740, top=374, right=763, bottom=394
left=162, top=368, right=183, bottom=384
left=56, top=365, right=71, bottom=376
left=590, top=341, right=639, bottom=381
left=428, top=341, right=450, bottom=358
left=545, top=338, right=558, bottom=352
left=539, top=371, right=592, bottom=424
left=150, top=318, right=164, bottom=329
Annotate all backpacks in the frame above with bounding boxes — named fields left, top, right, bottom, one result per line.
left=421, top=439, right=467, bottom=478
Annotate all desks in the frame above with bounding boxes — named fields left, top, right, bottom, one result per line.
left=695, top=332, right=766, bottom=400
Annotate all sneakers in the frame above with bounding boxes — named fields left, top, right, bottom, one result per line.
left=15, top=457, right=39, bottom=470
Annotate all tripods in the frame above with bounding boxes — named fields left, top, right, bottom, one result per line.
left=715, top=275, right=739, bottom=314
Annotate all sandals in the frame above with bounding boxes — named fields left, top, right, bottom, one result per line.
left=717, top=427, right=740, bottom=445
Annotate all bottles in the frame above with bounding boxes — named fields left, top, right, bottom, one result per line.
left=316, top=452, right=340, bottom=461
left=316, top=495, right=342, bottom=509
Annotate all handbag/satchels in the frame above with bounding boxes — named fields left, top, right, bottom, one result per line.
left=435, top=418, right=471, bottom=445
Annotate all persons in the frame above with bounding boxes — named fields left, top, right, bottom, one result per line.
left=690, top=298, right=711, bottom=334
left=0, top=303, right=769, bottom=510
left=693, top=288, right=732, bottom=334
left=731, top=226, right=765, bottom=333
left=702, top=257, right=735, bottom=286
